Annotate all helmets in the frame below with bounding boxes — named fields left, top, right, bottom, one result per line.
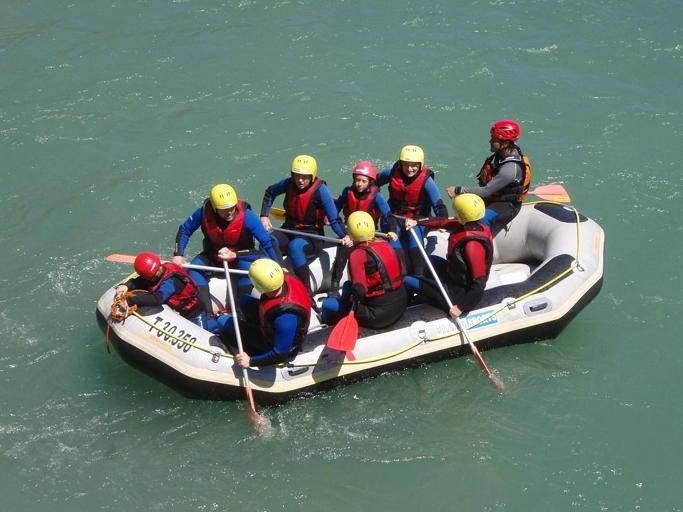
left=352, top=162, right=377, bottom=181
left=248, top=258, right=284, bottom=294
left=346, top=210, right=376, bottom=243
left=135, top=253, right=161, bottom=280
left=453, top=193, right=487, bottom=223
left=398, top=144, right=425, bottom=171
left=209, top=183, right=240, bottom=212
left=491, top=119, right=520, bottom=142
left=290, top=154, right=318, bottom=183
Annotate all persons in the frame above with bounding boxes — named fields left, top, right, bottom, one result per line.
left=113, top=252, right=208, bottom=333
left=319, top=209, right=407, bottom=328
left=217, top=246, right=311, bottom=369
left=167, top=182, right=282, bottom=313
left=448, top=119, right=530, bottom=242
left=330, top=161, right=398, bottom=302
left=404, top=193, right=495, bottom=320
left=380, top=143, right=449, bottom=277
left=262, top=152, right=354, bottom=301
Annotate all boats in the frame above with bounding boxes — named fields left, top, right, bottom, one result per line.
left=90, top=197, right=610, bottom=404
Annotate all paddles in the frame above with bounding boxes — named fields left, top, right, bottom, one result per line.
left=107, top=253, right=250, bottom=277
left=326, top=302, right=358, bottom=353
left=223, top=260, right=260, bottom=415
left=526, top=185, right=571, bottom=203
left=409, top=228, right=489, bottom=374
left=269, top=206, right=392, bottom=238
